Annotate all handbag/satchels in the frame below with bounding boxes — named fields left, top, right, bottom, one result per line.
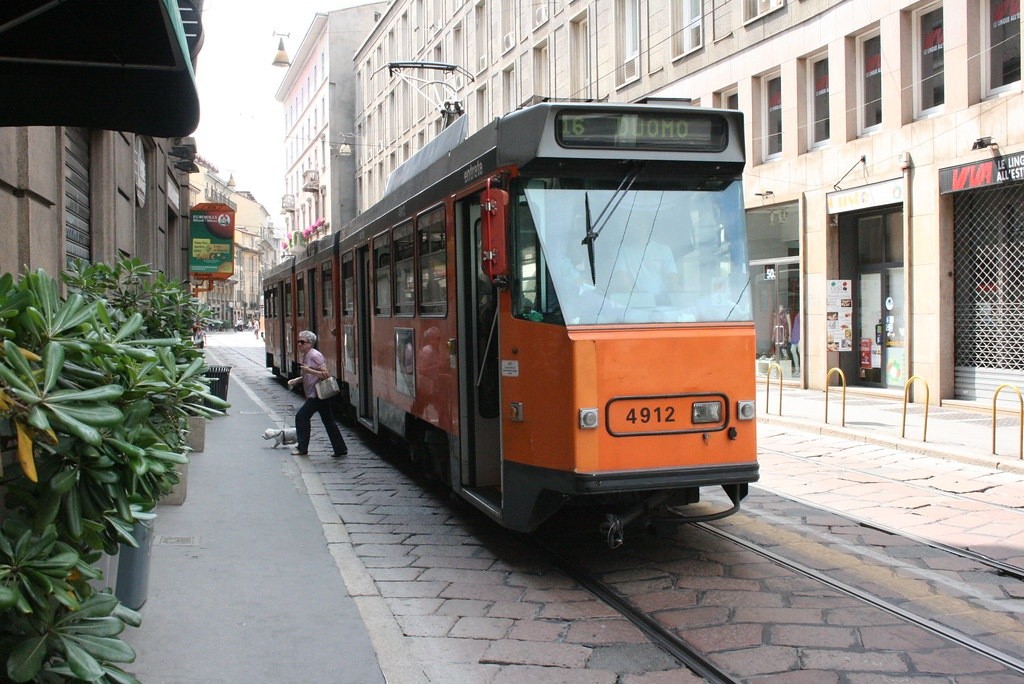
left=315, top=370, right=340, bottom=400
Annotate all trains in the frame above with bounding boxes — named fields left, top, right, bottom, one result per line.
left=264, top=62, right=759, bottom=571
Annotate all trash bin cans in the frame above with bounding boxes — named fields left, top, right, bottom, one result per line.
left=201, top=365, right=232, bottom=412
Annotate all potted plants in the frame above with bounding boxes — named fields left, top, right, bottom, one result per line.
left=0, top=250, right=230, bottom=684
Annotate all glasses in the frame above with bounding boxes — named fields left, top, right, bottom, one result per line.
left=298, top=340, right=310, bottom=345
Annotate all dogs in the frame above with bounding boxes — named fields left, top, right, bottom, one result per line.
left=261, top=428, right=299, bottom=449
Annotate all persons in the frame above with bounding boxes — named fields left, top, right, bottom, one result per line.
left=410, top=326, right=453, bottom=434
left=195, top=326, right=207, bottom=350
left=246, top=319, right=252, bottom=332
left=288, top=330, right=348, bottom=458
left=253, top=320, right=259, bottom=339
left=790, top=312, right=800, bottom=378
left=770, top=305, right=792, bottom=359
left=608, top=208, right=678, bottom=296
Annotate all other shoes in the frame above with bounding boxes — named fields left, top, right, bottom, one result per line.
left=330, top=451, right=348, bottom=457
left=290, top=450, right=308, bottom=455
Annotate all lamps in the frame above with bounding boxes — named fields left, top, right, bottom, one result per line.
left=340, top=134, right=351, bottom=155
left=272, top=31, right=291, bottom=67
left=227, top=173, right=236, bottom=186
left=972, top=137, right=997, bottom=150
left=168, top=146, right=199, bottom=173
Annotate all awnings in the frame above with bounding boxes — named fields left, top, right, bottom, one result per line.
left=1, top=0, right=200, bottom=138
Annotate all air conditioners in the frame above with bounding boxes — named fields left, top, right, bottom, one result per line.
left=480, top=54, right=488, bottom=71
left=456, top=73, right=463, bottom=89
left=624, top=55, right=638, bottom=82
left=503, top=32, right=515, bottom=50
left=533, top=4, right=547, bottom=26
